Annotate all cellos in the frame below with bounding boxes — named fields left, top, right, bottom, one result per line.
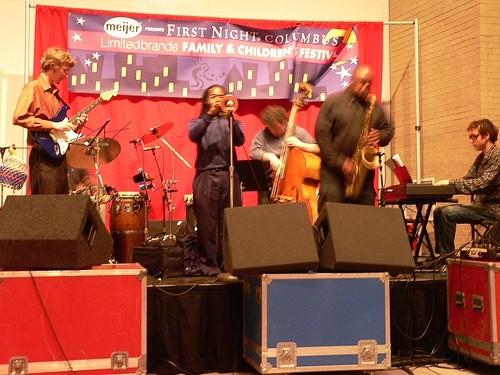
left=269, top=82, right=321, bottom=225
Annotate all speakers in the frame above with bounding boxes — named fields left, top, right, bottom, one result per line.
left=312, top=202, right=415, bottom=276
left=483, top=218, right=500, bottom=247
left=0, top=194, right=113, bottom=270
left=221, top=203, right=319, bottom=276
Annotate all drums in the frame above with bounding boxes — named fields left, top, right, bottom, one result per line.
left=109, top=192, right=147, bottom=264
left=75, top=185, right=114, bottom=205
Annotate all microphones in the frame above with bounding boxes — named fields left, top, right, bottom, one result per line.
left=144, top=146, right=160, bottom=150
left=226, top=101, right=234, bottom=106
left=129, top=139, right=140, bottom=143
left=372, top=152, right=385, bottom=156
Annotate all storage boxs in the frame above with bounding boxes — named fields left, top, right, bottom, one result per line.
left=243, top=273, right=393, bottom=373
left=447, top=257, right=500, bottom=366
left=0, top=262, right=147, bottom=375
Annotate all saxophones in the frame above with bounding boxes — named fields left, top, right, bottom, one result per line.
left=343, top=93, right=377, bottom=199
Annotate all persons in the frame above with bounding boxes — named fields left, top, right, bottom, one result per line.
left=188, top=84, right=245, bottom=276
left=12, top=47, right=88, bottom=195
left=67, top=165, right=97, bottom=195
left=417, top=119, right=500, bottom=276
left=248, top=105, right=320, bottom=205
left=314, top=65, right=396, bottom=215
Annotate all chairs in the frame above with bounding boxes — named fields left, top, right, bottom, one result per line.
left=470, top=220, right=500, bottom=260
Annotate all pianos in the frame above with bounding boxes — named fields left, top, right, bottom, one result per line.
left=378, top=183, right=456, bottom=268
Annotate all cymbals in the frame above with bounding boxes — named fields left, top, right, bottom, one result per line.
left=137, top=120, right=175, bottom=147
left=66, top=137, right=121, bottom=169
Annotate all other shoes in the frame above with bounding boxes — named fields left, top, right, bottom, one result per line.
left=422, top=260, right=447, bottom=269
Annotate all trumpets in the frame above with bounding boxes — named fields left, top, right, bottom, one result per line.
left=218, top=95, right=239, bottom=113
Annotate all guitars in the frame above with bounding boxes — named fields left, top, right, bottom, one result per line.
left=29, top=89, right=119, bottom=159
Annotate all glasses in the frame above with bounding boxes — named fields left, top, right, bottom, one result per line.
left=469, top=134, right=482, bottom=141
